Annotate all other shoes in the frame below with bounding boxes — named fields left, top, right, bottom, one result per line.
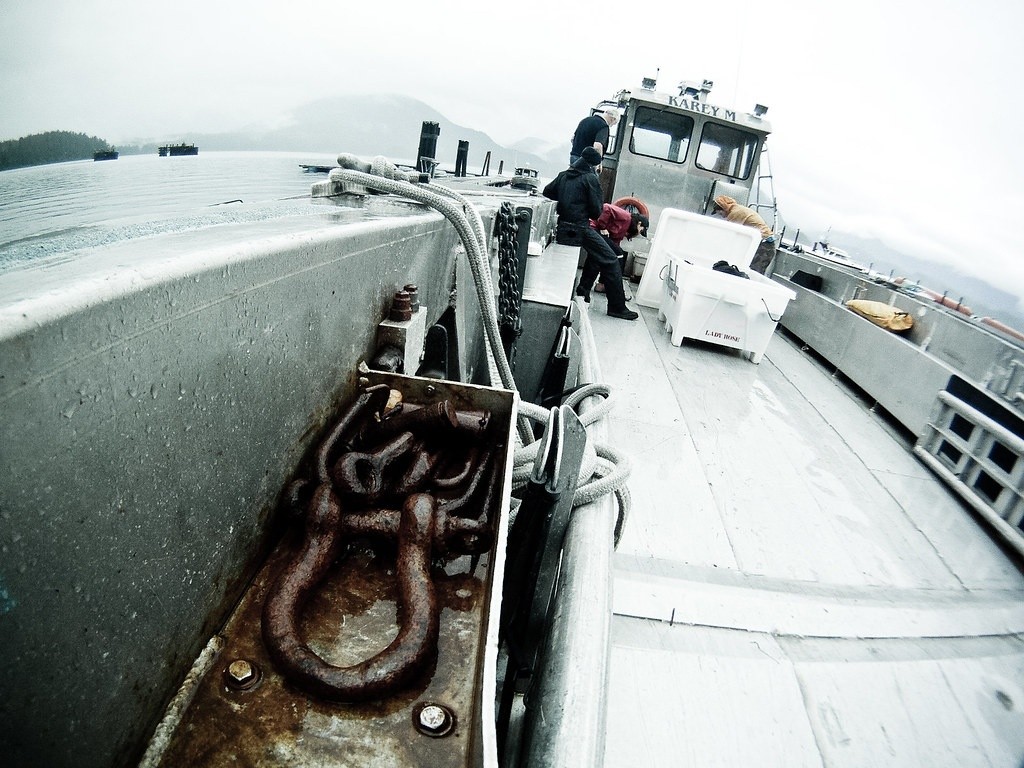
left=607, top=306, right=638, bottom=320
left=594, top=283, right=605, bottom=293
left=625, top=295, right=632, bottom=301
left=576, top=286, right=590, bottom=303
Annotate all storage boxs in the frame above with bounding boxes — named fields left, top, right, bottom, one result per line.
left=661, top=250, right=796, bottom=365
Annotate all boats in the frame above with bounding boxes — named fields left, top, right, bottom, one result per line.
left=91, top=146, right=116, bottom=161
left=158, top=145, right=197, bottom=157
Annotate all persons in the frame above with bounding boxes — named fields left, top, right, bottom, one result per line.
left=543, top=147, right=639, bottom=320
left=711, top=195, right=775, bottom=265
left=589, top=203, right=649, bottom=293
left=570, top=108, right=621, bottom=177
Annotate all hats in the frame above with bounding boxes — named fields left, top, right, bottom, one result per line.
left=581, top=147, right=601, bottom=166
left=639, top=214, right=649, bottom=237
